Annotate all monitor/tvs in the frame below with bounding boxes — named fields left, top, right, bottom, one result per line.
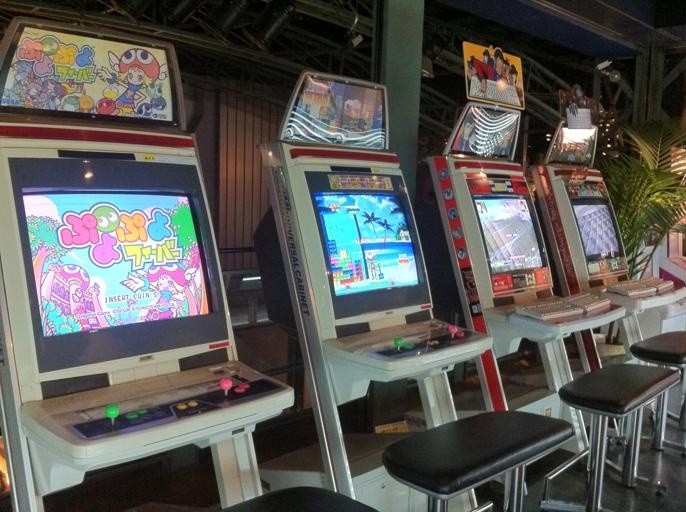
left=475, top=199, right=543, bottom=274
left=23, top=194, right=209, bottom=337
left=573, top=205, right=619, bottom=256
left=315, top=194, right=419, bottom=296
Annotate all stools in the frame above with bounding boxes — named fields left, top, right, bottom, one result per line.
left=556, top=361, right=680, bottom=511
left=628, top=331, right=685, bottom=429
left=380, top=407, right=576, bottom=512
left=216, top=484, right=381, bottom=512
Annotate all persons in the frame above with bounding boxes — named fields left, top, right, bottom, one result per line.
left=467, top=49, right=522, bottom=100
